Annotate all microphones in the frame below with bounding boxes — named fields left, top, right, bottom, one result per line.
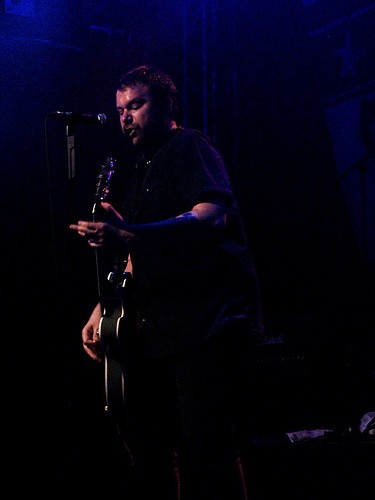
left=54, top=111, right=107, bottom=126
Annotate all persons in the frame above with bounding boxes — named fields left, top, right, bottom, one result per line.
left=69, top=65, right=265, bottom=499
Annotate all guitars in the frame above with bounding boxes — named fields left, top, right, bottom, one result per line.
left=89, top=155, right=141, bottom=426
